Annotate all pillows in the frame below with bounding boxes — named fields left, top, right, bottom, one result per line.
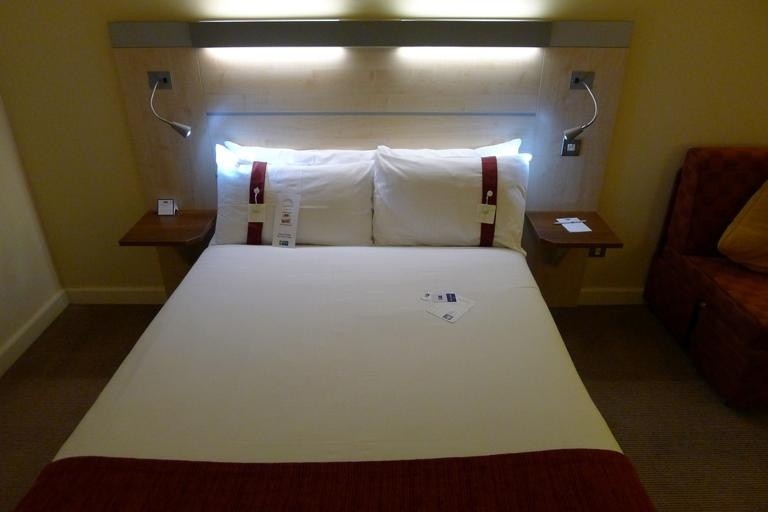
left=714, top=178, right=768, bottom=273
left=213, top=139, right=534, bottom=253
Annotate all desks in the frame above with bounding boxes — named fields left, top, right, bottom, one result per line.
left=117, top=208, right=217, bottom=264
left=526, top=212, right=625, bottom=267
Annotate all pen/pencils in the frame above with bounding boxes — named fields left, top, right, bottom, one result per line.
left=552, top=220, right=589, bottom=225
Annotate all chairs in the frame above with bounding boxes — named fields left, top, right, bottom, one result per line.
left=639, top=143, right=767, bottom=417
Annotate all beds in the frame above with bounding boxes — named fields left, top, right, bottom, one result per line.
left=14, top=237, right=662, bottom=512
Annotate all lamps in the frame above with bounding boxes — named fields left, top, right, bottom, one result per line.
left=146, top=69, right=192, bottom=139
left=562, top=70, right=598, bottom=144
